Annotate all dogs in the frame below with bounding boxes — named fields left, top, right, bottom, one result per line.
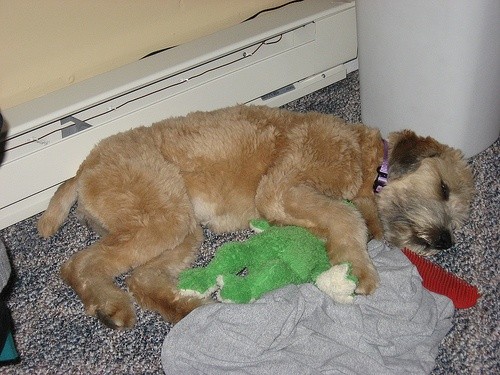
left=37, top=103, right=476, bottom=330
